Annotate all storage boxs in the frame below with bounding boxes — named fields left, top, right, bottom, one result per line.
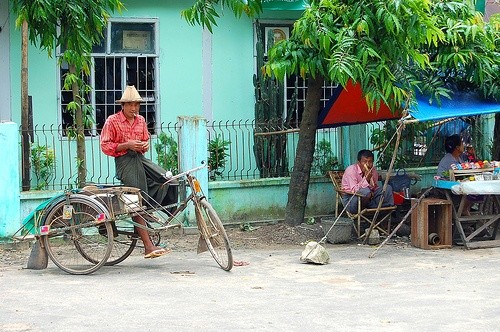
left=321, top=216, right=354, bottom=244
left=412, top=199, right=452, bottom=249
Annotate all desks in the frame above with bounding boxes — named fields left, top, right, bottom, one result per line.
left=432, top=179, right=500, bottom=249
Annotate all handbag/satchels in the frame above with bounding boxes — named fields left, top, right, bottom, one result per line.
left=387, top=168, right=411, bottom=192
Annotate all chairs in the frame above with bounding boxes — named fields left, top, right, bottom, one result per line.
left=328, top=170, right=397, bottom=239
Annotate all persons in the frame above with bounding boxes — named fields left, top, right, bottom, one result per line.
left=341, top=149, right=394, bottom=214
left=100, top=85, right=180, bottom=259
left=437, top=135, right=484, bottom=216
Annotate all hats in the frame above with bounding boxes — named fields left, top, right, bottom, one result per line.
left=116, top=85, right=145, bottom=102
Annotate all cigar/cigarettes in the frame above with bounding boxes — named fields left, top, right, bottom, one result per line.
left=132, top=112, right=137, bottom=116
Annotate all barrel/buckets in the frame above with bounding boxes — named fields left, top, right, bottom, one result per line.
left=388, top=168, right=411, bottom=192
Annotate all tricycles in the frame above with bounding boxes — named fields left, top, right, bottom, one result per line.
left=27, top=161, right=233, bottom=274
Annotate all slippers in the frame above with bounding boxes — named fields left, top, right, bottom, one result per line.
left=143, top=213, right=161, bottom=222
left=144, top=247, right=170, bottom=259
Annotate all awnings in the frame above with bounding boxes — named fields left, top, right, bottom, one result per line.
left=315, top=67, right=500, bottom=245
